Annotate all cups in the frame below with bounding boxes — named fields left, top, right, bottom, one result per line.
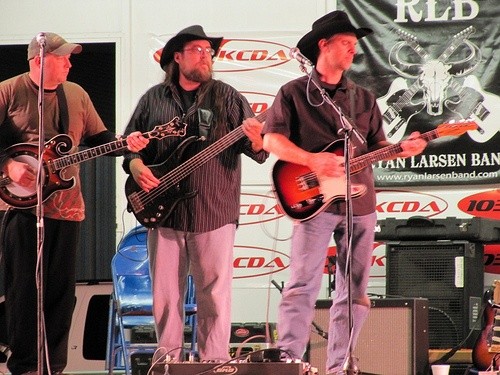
left=431, top=365, right=450, bottom=375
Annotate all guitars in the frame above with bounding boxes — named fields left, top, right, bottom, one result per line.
left=271, top=120, right=478, bottom=224
left=0, top=115, right=188, bottom=209
left=470, top=279, right=500, bottom=371
left=124, top=106, right=273, bottom=230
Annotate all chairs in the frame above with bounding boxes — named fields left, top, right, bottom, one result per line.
left=104, top=224, right=198, bottom=375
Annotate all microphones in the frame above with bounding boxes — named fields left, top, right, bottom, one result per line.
left=289, top=48, right=315, bottom=68
left=36, top=32, right=47, bottom=46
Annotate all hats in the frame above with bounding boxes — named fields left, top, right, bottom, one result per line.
left=296, top=10, right=373, bottom=58
left=160, top=25, right=223, bottom=72
left=28, top=32, right=82, bottom=62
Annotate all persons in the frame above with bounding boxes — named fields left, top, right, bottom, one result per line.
left=0, top=33, right=150, bottom=375
left=260, top=11, right=428, bottom=375
left=123, top=26, right=270, bottom=362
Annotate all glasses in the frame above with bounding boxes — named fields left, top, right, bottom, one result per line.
left=179, top=45, right=215, bottom=56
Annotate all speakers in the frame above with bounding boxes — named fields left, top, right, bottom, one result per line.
left=385, top=240, right=484, bottom=350
left=300, top=296, right=430, bottom=375
left=152, top=360, right=304, bottom=375
